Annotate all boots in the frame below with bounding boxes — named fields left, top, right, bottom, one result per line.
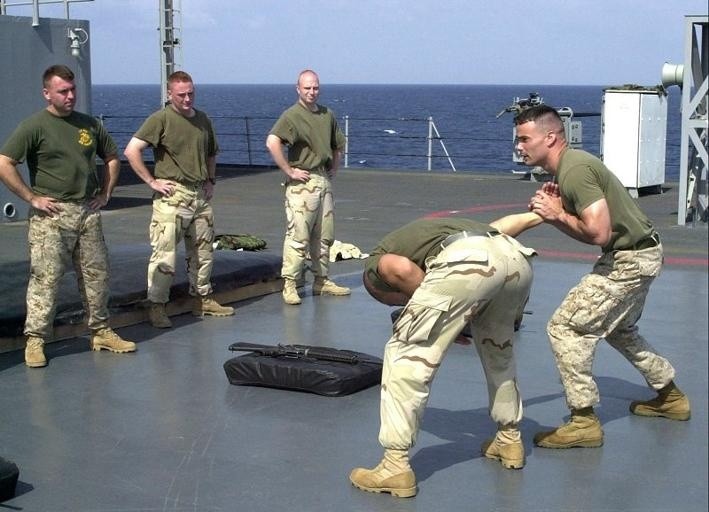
left=348, top=449, right=417, bottom=498
left=533, top=405, right=603, bottom=450
left=148, top=303, right=173, bottom=328
left=481, top=423, right=525, bottom=469
left=89, top=327, right=137, bottom=353
left=191, top=293, right=235, bottom=317
left=24, top=334, right=47, bottom=368
left=630, top=381, right=691, bottom=423
left=311, top=275, right=351, bottom=296
left=281, top=278, right=302, bottom=305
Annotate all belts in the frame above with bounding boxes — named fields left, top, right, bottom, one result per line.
left=310, top=166, right=328, bottom=172
left=429, top=232, right=501, bottom=257
left=619, top=235, right=659, bottom=253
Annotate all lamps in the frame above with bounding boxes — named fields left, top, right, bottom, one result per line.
left=67, top=27, right=88, bottom=56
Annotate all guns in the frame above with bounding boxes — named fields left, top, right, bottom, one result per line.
left=229, top=341, right=382, bottom=364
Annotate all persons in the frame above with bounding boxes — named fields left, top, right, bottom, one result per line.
left=0, top=64, right=136, bottom=368
left=349, top=182, right=562, bottom=499
left=266, top=69, right=351, bottom=305
left=123, top=71, right=234, bottom=329
left=513, top=104, right=689, bottom=450
